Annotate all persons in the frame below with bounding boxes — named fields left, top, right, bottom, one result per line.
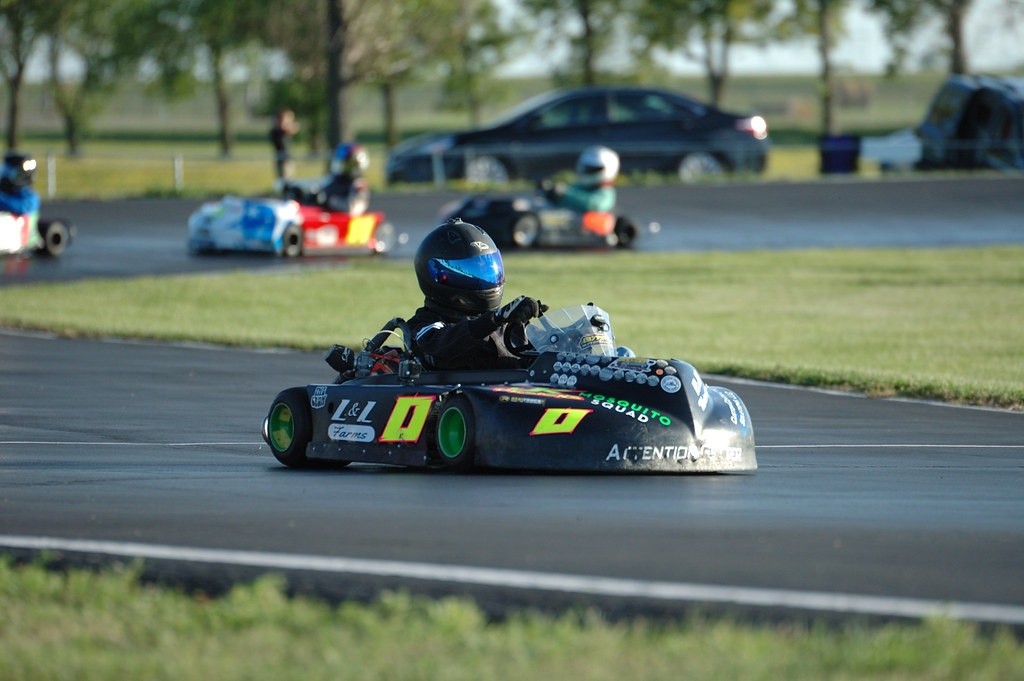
left=410, top=226, right=603, bottom=383
left=282, top=147, right=370, bottom=215
left=267, top=108, right=308, bottom=183
left=1, top=152, right=40, bottom=226
left=538, top=147, right=621, bottom=219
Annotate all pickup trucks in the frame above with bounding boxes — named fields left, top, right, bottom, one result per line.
left=816, top=76, right=1024, bottom=175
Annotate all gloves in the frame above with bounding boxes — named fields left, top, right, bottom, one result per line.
left=493, top=294, right=538, bottom=324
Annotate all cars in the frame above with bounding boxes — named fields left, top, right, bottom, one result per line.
left=383, top=80, right=773, bottom=187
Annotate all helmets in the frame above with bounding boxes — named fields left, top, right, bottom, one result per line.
left=328, top=142, right=370, bottom=178
left=1, top=150, right=35, bottom=187
left=575, top=145, right=621, bottom=187
left=413, top=218, right=506, bottom=321
